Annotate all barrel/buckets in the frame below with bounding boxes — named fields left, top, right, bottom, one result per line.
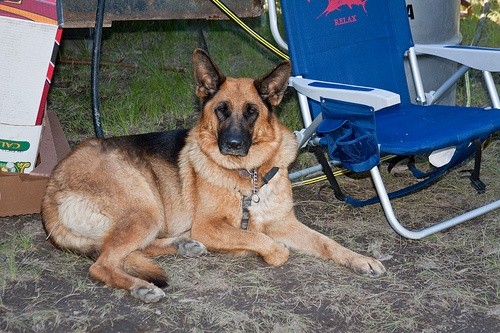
left=404, top=0, right=462, bottom=106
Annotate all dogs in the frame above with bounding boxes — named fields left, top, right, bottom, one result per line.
left=40, top=45, right=387, bottom=304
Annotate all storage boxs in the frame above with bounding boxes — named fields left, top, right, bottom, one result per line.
left=1, top=0, right=64, bottom=173
left=1, top=109, right=73, bottom=218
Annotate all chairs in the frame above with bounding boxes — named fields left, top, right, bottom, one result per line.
left=268, top=0, right=500, bottom=240
